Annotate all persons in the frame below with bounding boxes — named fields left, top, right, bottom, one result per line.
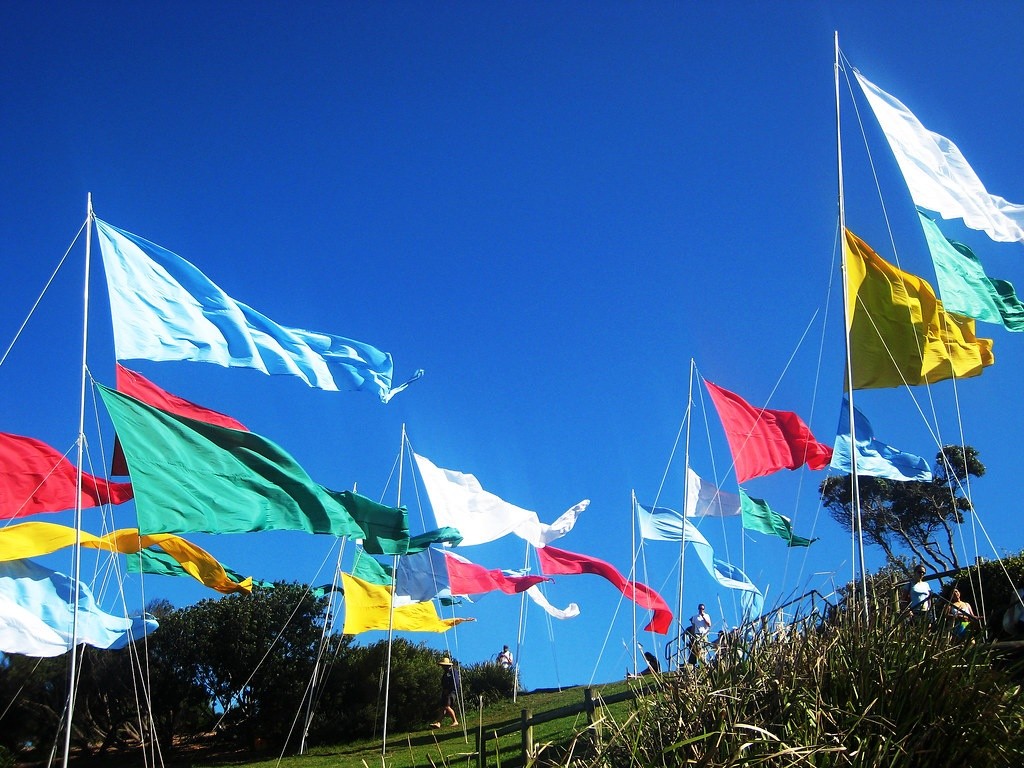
left=493, top=644, right=518, bottom=682
left=680, top=607, right=696, bottom=668
left=715, top=627, right=754, bottom=664
left=430, top=657, right=461, bottom=733
left=950, top=587, right=980, bottom=645
left=626, top=651, right=665, bottom=682
left=694, top=602, right=711, bottom=666
left=898, top=564, right=940, bottom=643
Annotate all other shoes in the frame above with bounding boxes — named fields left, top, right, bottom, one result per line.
left=430, top=723, right=441, bottom=728
left=451, top=722, right=458, bottom=726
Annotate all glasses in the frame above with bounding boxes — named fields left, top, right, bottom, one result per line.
left=917, top=570, right=926, bottom=574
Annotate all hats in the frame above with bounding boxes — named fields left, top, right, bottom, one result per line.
left=437, top=658, right=453, bottom=666
left=504, top=645, right=509, bottom=649
